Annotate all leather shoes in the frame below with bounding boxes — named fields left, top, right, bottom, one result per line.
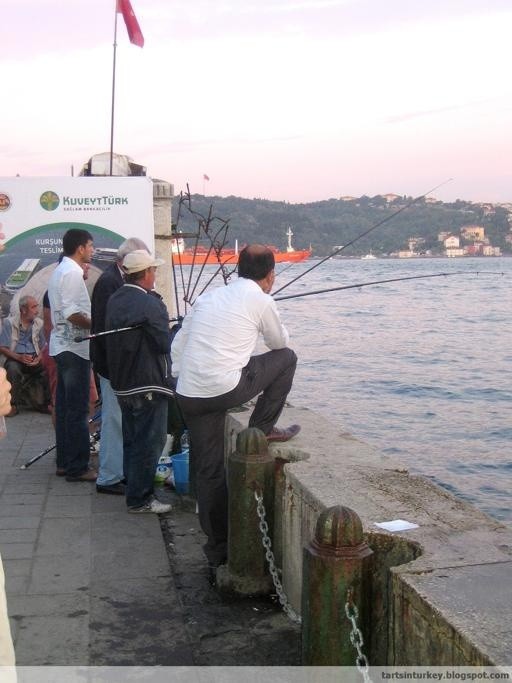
left=265, top=423, right=301, bottom=442
left=96, top=480, right=128, bottom=496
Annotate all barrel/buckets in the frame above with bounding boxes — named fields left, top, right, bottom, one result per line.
left=153, top=434, right=174, bottom=486
left=170, top=454, right=189, bottom=493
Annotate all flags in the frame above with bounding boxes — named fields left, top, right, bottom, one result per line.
left=115, top=0, right=151, bottom=47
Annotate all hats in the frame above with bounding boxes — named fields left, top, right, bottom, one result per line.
left=120, top=247, right=164, bottom=276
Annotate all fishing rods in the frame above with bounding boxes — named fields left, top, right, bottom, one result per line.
left=20, top=250, right=314, bottom=469
left=270, top=178, right=454, bottom=294
left=73, top=271, right=509, bottom=344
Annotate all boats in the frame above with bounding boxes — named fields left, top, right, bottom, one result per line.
left=361, top=252, right=376, bottom=259
left=93, top=246, right=118, bottom=263
left=171, top=226, right=311, bottom=266
left=4, top=257, right=41, bottom=292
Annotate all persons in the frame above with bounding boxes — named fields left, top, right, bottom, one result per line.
left=86, top=236, right=151, bottom=497
left=1, top=247, right=115, bottom=445
left=100, top=248, right=184, bottom=517
left=49, top=228, right=103, bottom=482
left=0, top=367, right=21, bottom=677
left=169, top=243, right=305, bottom=583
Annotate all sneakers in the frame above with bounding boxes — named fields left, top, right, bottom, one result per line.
left=55, top=465, right=94, bottom=476
left=6, top=403, right=20, bottom=418
left=65, top=470, right=98, bottom=481
left=127, top=499, right=173, bottom=514
